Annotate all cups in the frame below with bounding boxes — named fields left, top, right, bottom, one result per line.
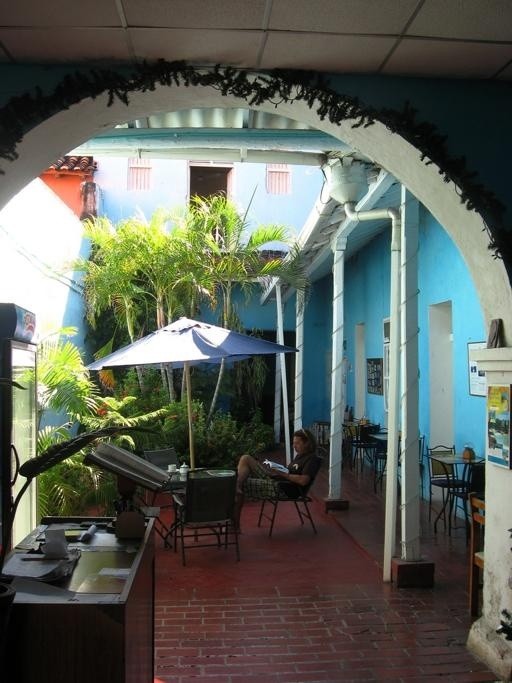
left=168, top=463, right=177, bottom=471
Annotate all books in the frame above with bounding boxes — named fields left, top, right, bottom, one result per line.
left=263, top=459, right=289, bottom=473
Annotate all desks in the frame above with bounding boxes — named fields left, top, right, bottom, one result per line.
left=0, top=515, right=156, bottom=683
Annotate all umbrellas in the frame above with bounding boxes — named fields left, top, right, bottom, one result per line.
left=80, top=312, right=303, bottom=471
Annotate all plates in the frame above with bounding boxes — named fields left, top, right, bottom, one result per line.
left=166, top=469, right=179, bottom=473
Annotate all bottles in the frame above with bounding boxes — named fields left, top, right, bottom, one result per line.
left=179, top=474, right=187, bottom=482
left=358, top=415, right=368, bottom=424
left=179, top=461, right=189, bottom=474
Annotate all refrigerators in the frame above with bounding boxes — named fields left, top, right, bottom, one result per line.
left=0, top=302, right=37, bottom=550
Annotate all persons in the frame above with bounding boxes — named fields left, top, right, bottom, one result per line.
left=225, top=428, right=321, bottom=534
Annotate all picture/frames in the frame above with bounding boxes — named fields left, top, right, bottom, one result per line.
left=467, top=341, right=487, bottom=396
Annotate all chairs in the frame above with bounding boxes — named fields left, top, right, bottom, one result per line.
left=313, top=413, right=485, bottom=535
left=119, top=445, right=323, bottom=567
left=466, top=495, right=486, bottom=610
